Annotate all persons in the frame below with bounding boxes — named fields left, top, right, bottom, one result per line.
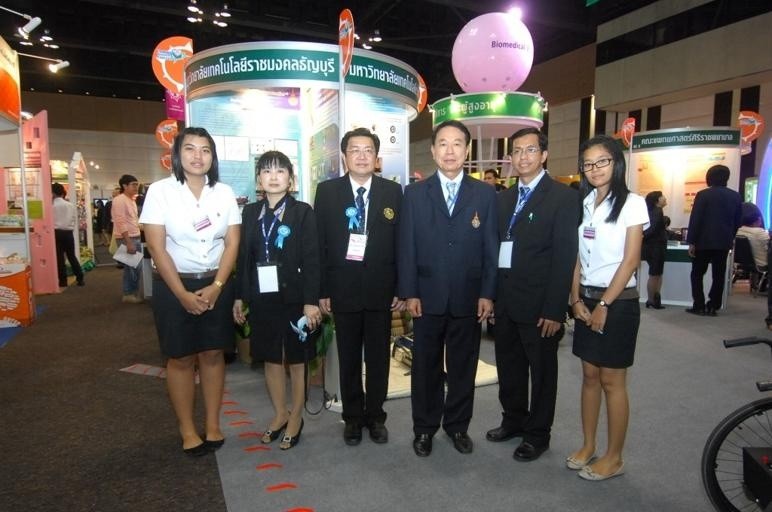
left=737, top=202, right=772, bottom=271
left=315, top=127, right=403, bottom=447
left=486, top=126, right=583, bottom=461
left=395, top=120, right=500, bottom=454
left=483, top=169, right=507, bottom=192
left=234, top=150, right=323, bottom=449
left=96, top=174, right=151, bottom=303
left=137, top=126, right=243, bottom=456
left=685, top=166, right=743, bottom=316
left=643, top=191, right=670, bottom=310
left=565, top=133, right=650, bottom=480
left=53, top=183, right=85, bottom=290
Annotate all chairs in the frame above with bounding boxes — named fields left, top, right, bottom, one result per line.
left=730, top=234, right=772, bottom=296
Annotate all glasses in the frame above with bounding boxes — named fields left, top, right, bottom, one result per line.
left=579, top=157, right=614, bottom=173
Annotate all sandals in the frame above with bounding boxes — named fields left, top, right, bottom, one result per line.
left=260, top=411, right=291, bottom=444
left=280, top=417, right=304, bottom=450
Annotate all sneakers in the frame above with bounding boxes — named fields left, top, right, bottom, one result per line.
left=122, top=294, right=145, bottom=304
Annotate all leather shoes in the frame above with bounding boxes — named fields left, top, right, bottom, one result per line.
left=513, top=440, right=549, bottom=462
left=413, top=433, right=432, bottom=457
left=486, top=426, right=523, bottom=442
left=685, top=308, right=705, bottom=316
left=344, top=422, right=362, bottom=445
left=203, top=438, right=224, bottom=452
left=446, top=426, right=473, bottom=453
left=184, top=442, right=206, bottom=456
left=367, top=418, right=387, bottom=442
left=566, top=446, right=597, bottom=470
left=707, top=308, right=715, bottom=316
left=578, top=459, right=626, bottom=481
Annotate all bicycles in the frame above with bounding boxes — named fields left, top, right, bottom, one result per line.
left=701, top=336, right=772, bottom=512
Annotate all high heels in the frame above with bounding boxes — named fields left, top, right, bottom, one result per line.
left=646, top=301, right=665, bottom=309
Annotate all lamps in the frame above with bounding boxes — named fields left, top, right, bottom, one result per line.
left=10, top=47, right=74, bottom=75
left=0, top=3, right=63, bottom=53
left=184, top=0, right=234, bottom=31
left=351, top=30, right=385, bottom=53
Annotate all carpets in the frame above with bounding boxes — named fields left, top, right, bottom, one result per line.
left=201, top=278, right=770, bottom=511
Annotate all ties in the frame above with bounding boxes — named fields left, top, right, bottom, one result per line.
left=355, top=187, right=366, bottom=234
left=445, top=180, right=456, bottom=216
left=519, top=186, right=530, bottom=205
